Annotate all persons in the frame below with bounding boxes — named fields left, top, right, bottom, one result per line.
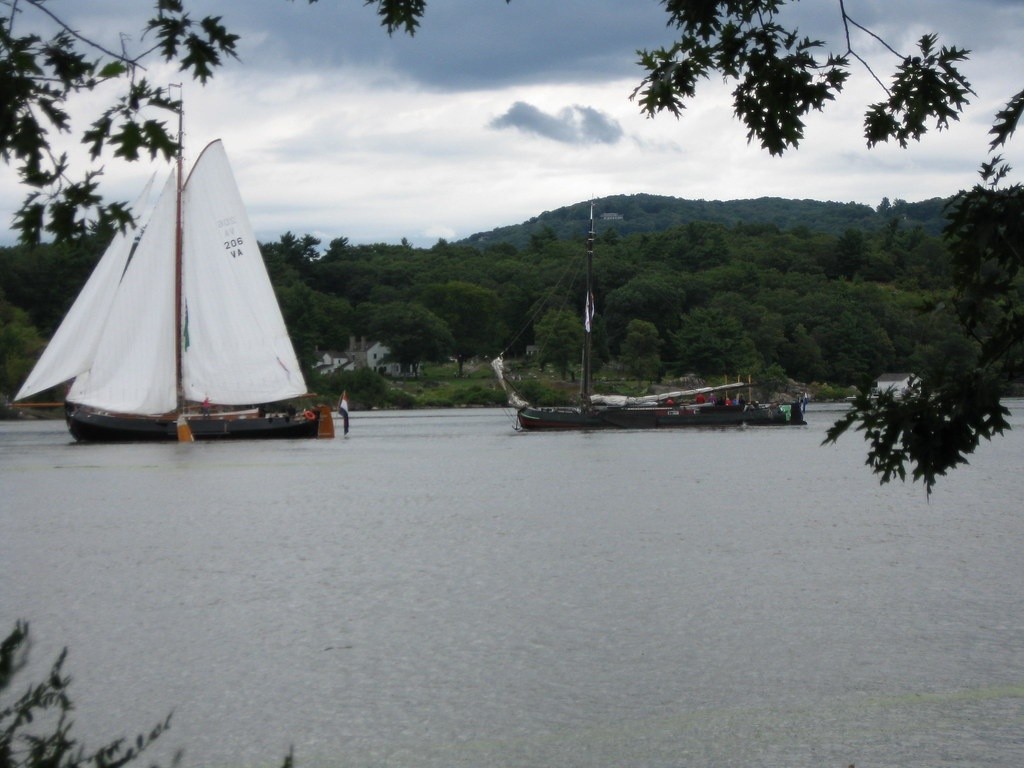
left=288, top=403, right=296, bottom=423
left=707, top=392, right=717, bottom=401
left=696, top=391, right=706, bottom=409
left=725, top=398, right=732, bottom=405
left=203, top=396, right=210, bottom=420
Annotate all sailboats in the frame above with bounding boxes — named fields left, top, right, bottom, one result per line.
left=491, top=194, right=808, bottom=432
left=5, top=137, right=335, bottom=441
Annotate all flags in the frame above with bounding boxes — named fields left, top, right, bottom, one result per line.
left=337, top=390, right=348, bottom=417
